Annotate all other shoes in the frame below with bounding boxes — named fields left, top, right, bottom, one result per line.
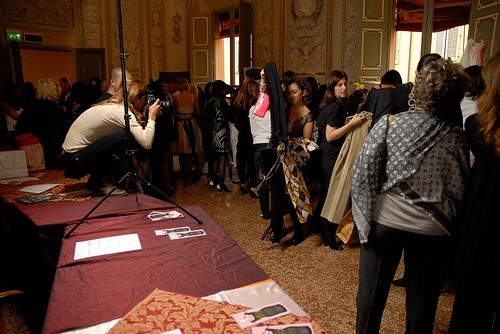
left=325, top=233, right=341, bottom=250
left=393, top=277, right=406, bottom=287
left=217, top=186, right=232, bottom=193
left=291, top=232, right=304, bottom=243
left=272, top=232, right=284, bottom=242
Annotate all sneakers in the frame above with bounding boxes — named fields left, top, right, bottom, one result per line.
left=91, top=179, right=128, bottom=196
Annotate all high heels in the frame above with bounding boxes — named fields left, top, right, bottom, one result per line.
left=240, top=186, right=249, bottom=195
left=249, top=191, right=261, bottom=199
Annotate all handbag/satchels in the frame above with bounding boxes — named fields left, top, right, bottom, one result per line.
left=337, top=209, right=361, bottom=245
left=14, top=133, right=46, bottom=172
left=0, top=149, right=29, bottom=179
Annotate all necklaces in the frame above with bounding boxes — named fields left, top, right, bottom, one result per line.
left=291, top=104, right=306, bottom=112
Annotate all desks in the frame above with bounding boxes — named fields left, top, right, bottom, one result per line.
left=41, top=204, right=329, bottom=334
left=1, top=165, right=178, bottom=273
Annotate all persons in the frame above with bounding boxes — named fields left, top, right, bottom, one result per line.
left=0, top=78, right=110, bottom=170
left=350, top=59, right=469, bottom=334
left=96, top=39, right=500, bottom=288
left=61, top=80, right=162, bottom=194
left=447, top=52, right=500, bottom=334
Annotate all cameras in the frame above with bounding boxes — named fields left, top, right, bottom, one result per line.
left=145, top=90, right=171, bottom=109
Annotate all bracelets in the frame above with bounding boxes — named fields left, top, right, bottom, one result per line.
left=259, top=82, right=266, bottom=93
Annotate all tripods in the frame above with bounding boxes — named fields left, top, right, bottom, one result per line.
left=64, top=0, right=203, bottom=240
